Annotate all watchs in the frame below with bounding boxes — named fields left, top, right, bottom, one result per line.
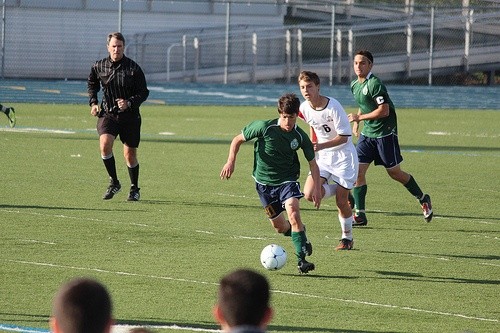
left=127, top=100, right=131, bottom=107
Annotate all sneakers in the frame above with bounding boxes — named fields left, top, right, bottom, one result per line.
left=294, top=253, right=316, bottom=274
left=334, top=238, right=354, bottom=250
left=7, top=106, right=16, bottom=128
left=348, top=190, right=355, bottom=209
left=127, top=184, right=140, bottom=201
left=301, top=231, right=312, bottom=256
left=351, top=215, right=367, bottom=226
left=103, top=180, right=121, bottom=199
left=420, top=194, right=433, bottom=223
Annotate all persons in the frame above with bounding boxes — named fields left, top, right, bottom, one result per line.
left=48, top=276, right=115, bottom=333
left=87, top=32, right=149, bottom=201
left=0, top=104, right=16, bottom=128
left=214, top=266, right=274, bottom=333
left=220, top=93, right=322, bottom=273
left=347, top=51, right=433, bottom=226
left=295, top=70, right=357, bottom=251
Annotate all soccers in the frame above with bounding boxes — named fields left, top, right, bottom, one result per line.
left=260, top=244, right=287, bottom=270
